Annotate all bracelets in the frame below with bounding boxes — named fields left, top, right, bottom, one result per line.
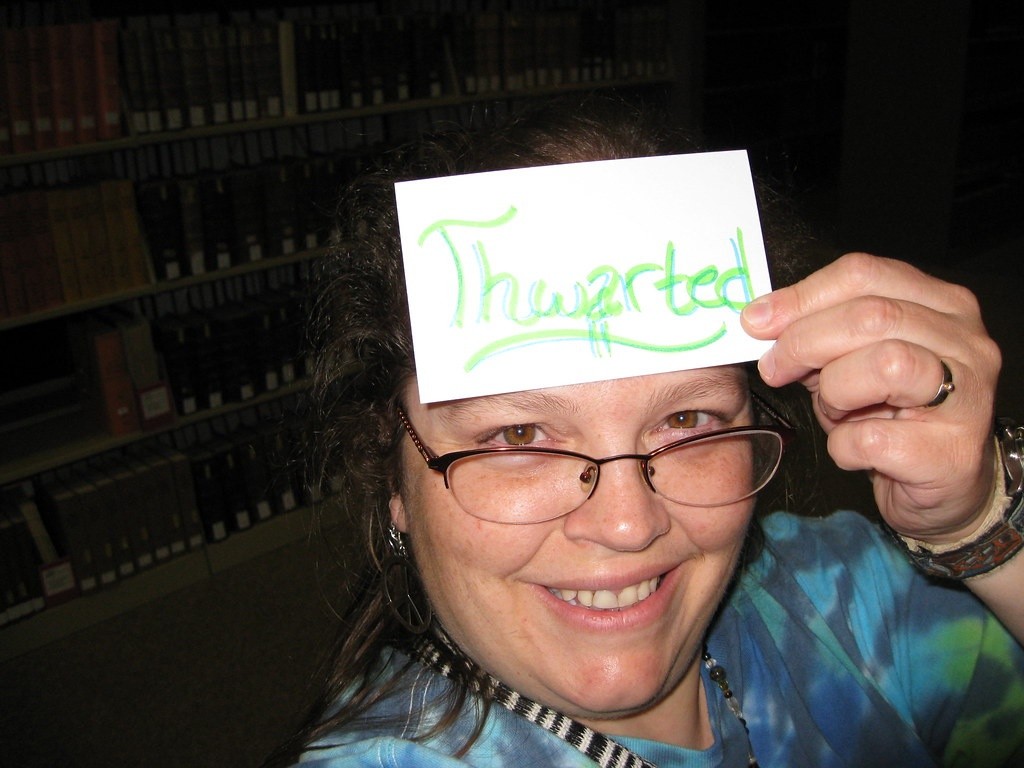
left=885, top=409, right=1024, bottom=578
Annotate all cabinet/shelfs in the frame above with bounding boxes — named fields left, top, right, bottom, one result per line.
left=0, top=73, right=677, bottom=665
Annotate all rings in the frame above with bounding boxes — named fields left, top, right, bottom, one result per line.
left=929, top=358, right=957, bottom=409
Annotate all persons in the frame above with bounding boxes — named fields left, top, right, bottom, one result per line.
left=295, top=107, right=1024, bottom=768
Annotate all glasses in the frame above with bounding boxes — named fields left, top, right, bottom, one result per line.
left=395, top=394, right=798, bottom=524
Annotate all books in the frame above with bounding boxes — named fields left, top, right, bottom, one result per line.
left=1, top=0, right=704, bottom=631
